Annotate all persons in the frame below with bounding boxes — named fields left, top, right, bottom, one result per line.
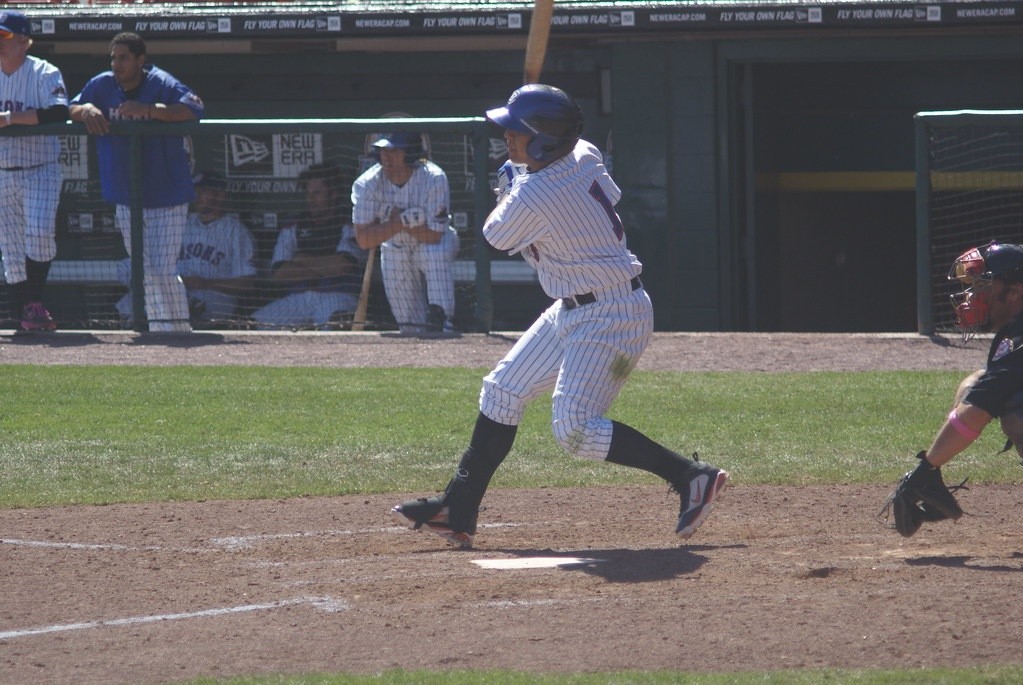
left=0, top=11, right=68, bottom=330
left=389, top=84, right=731, bottom=547
left=68, top=31, right=204, bottom=332
left=177, top=173, right=261, bottom=321
left=351, top=131, right=461, bottom=335
left=248, top=163, right=360, bottom=324
left=895, top=243, right=1023, bottom=538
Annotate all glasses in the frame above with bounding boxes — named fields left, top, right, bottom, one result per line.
left=0, top=30, right=28, bottom=44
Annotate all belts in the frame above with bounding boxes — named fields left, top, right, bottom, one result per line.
left=562, top=276, right=641, bottom=310
left=0, top=163, right=43, bottom=171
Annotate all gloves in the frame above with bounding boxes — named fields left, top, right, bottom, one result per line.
left=493, top=158, right=528, bottom=201
left=399, top=207, right=426, bottom=228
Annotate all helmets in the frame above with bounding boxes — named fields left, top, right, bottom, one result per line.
left=371, top=131, right=425, bottom=164
left=485, top=83, right=584, bottom=162
left=983, top=243, right=1023, bottom=287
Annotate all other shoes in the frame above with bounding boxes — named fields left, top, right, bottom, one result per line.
left=427, top=304, right=446, bottom=334
left=21, top=301, right=57, bottom=331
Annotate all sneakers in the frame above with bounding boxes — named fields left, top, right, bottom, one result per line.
left=391, top=493, right=479, bottom=548
left=666, top=451, right=731, bottom=539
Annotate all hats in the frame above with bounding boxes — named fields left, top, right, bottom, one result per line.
left=0, top=10, right=32, bottom=36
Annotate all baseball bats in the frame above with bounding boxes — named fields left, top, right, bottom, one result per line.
left=522, top=0, right=556, bottom=88
left=351, top=246, right=378, bottom=330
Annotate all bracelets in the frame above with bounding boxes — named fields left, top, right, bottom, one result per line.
left=6, top=111, right=10, bottom=125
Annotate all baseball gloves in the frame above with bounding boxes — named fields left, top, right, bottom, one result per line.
left=872, top=447, right=972, bottom=539
